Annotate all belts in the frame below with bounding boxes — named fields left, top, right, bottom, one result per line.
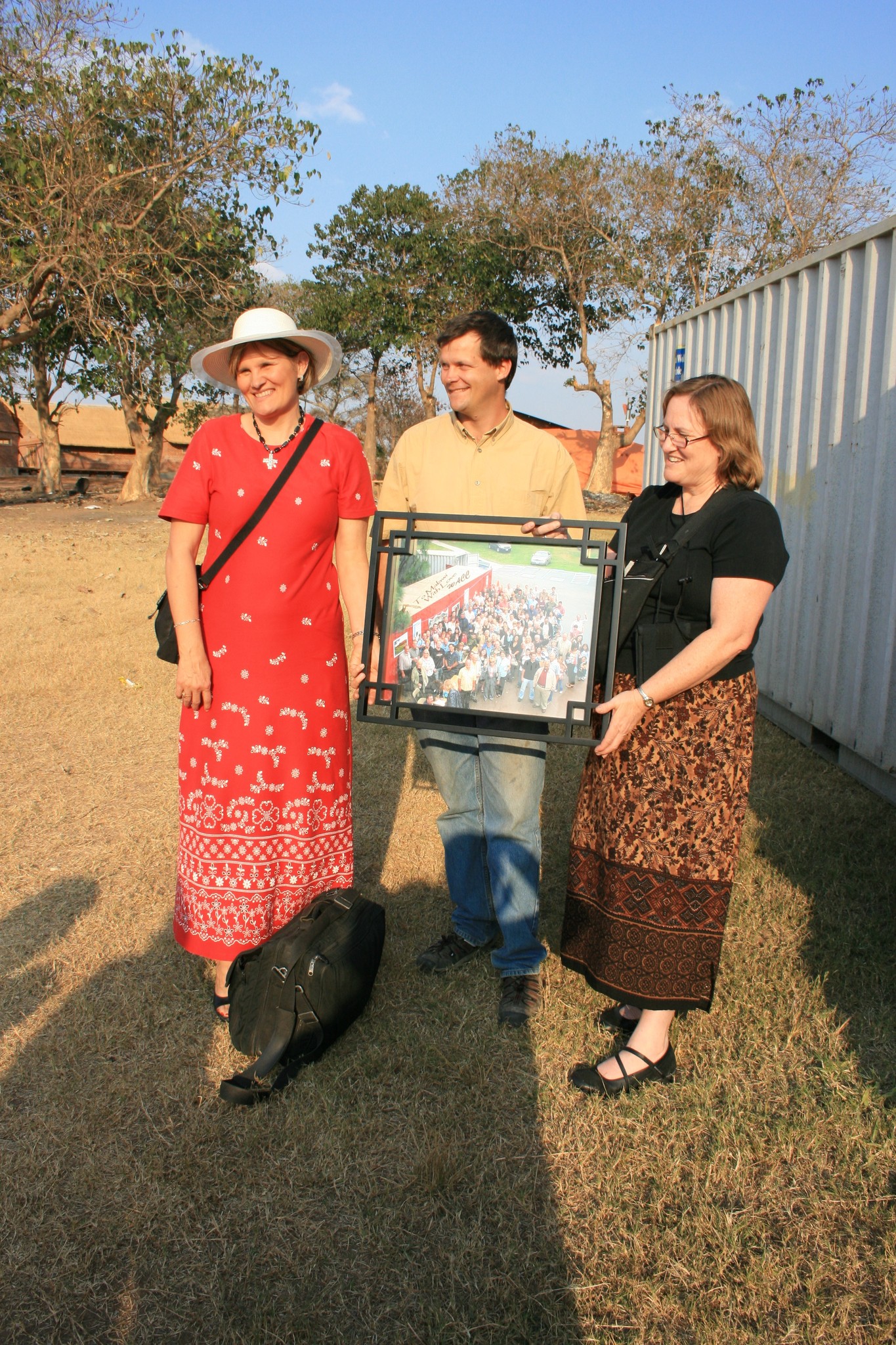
left=538, top=684, right=545, bottom=688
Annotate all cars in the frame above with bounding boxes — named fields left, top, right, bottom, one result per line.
left=530, top=550, right=552, bottom=566
left=488, top=542, right=512, bottom=553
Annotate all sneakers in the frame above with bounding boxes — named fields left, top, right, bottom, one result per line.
left=416, top=930, right=498, bottom=975
left=497, top=973, right=543, bottom=1027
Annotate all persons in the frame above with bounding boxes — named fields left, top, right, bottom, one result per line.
left=399, top=581, right=590, bottom=713
left=521, top=373, right=790, bottom=1097
left=366, top=310, right=587, bottom=1032
left=156, top=306, right=380, bottom=1022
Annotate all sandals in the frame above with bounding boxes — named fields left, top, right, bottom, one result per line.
left=213, top=993, right=231, bottom=1024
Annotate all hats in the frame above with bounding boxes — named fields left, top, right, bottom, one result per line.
left=190, top=308, right=342, bottom=397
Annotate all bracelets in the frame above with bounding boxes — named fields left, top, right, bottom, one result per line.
left=173, top=618, right=201, bottom=628
left=352, top=630, right=381, bottom=642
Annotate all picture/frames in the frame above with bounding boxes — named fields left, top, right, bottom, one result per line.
left=359, top=509, right=629, bottom=753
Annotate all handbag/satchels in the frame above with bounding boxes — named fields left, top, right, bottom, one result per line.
left=593, top=537, right=678, bottom=687
left=217, top=885, right=386, bottom=1104
left=148, top=564, right=201, bottom=664
left=511, top=655, right=518, bottom=666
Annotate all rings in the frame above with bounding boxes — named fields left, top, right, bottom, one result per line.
left=181, top=691, right=192, bottom=698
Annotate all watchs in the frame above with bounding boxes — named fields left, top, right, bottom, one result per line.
left=637, top=687, right=656, bottom=709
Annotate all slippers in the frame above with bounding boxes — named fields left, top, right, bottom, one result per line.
left=566, top=683, right=573, bottom=688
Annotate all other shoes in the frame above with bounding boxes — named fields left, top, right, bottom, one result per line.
left=542, top=709, right=545, bottom=713
left=554, top=689, right=557, bottom=692
left=533, top=705, right=540, bottom=707
left=530, top=699, right=533, bottom=703
left=496, top=694, right=500, bottom=697
left=578, top=677, right=586, bottom=681
left=559, top=691, right=563, bottom=693
left=518, top=698, right=522, bottom=702
left=572, top=1040, right=676, bottom=1098
left=518, top=686, right=520, bottom=688
left=484, top=698, right=487, bottom=701
left=491, top=699, right=493, bottom=701
left=473, top=699, right=477, bottom=703
left=599, top=1004, right=688, bottom=1033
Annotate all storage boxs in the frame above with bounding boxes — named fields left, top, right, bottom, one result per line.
left=647, top=215, right=896, bottom=808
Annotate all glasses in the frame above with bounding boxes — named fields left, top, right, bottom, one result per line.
left=653, top=424, right=711, bottom=448
left=490, top=661, right=494, bottom=663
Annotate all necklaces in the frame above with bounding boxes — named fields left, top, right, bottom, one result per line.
left=252, top=405, right=305, bottom=470
